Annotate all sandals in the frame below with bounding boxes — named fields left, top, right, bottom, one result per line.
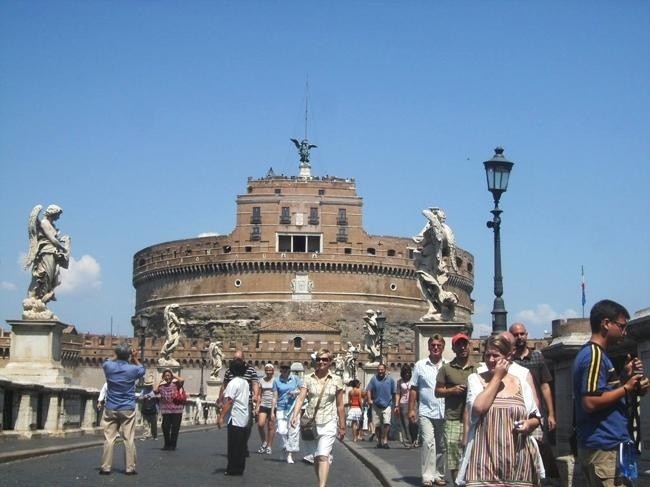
left=425, top=479, right=448, bottom=486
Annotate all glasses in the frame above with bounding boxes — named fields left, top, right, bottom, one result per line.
left=611, top=320, right=626, bottom=330
left=456, top=341, right=468, bottom=346
left=316, top=357, right=328, bottom=362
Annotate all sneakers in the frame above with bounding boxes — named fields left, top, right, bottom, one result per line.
left=377, top=443, right=389, bottom=448
left=126, top=468, right=136, bottom=475
left=257, top=444, right=333, bottom=464
left=100, top=467, right=111, bottom=474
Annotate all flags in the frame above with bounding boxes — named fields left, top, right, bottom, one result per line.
left=580, top=263, right=587, bottom=306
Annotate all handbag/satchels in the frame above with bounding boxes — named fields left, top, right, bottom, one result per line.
left=142, top=396, right=156, bottom=414
left=173, top=386, right=187, bottom=401
left=300, top=416, right=317, bottom=440
left=568, top=434, right=576, bottom=457
left=526, top=412, right=560, bottom=478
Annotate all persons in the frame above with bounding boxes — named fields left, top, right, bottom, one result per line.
left=411, top=206, right=461, bottom=316
left=361, top=309, right=382, bottom=363
left=96, top=322, right=650, bottom=487
left=27, top=204, right=70, bottom=308
left=160, top=303, right=185, bottom=360
left=569, top=299, right=649, bottom=486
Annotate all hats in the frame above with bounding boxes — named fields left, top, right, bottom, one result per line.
left=144, top=380, right=154, bottom=386
left=280, top=361, right=291, bottom=369
left=452, top=333, right=469, bottom=346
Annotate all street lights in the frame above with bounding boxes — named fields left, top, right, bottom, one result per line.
left=138, top=310, right=151, bottom=393
left=353, top=348, right=359, bottom=380
left=199, top=347, right=209, bottom=400
left=482, top=146, right=513, bottom=335
left=376, top=315, right=387, bottom=366
